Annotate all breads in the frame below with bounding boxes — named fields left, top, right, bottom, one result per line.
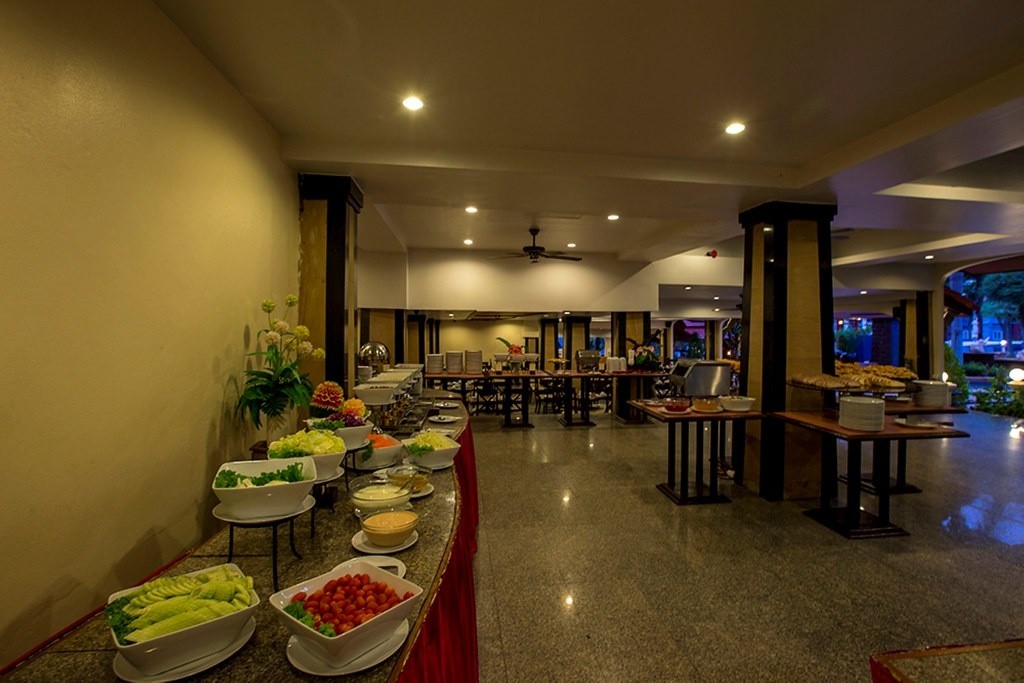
left=716, top=357, right=740, bottom=370
left=792, top=360, right=918, bottom=388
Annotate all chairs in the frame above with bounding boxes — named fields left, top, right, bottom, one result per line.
left=467, top=379, right=609, bottom=415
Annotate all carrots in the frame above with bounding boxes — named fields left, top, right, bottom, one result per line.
left=367, top=434, right=398, bottom=447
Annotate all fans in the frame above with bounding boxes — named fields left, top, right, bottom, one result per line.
left=486, top=225, right=582, bottom=264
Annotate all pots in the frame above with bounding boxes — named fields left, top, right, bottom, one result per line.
left=912, top=380, right=947, bottom=407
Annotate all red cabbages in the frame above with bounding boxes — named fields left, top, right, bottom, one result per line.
left=327, top=411, right=364, bottom=426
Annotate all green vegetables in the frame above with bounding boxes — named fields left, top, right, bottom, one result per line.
left=284, top=600, right=333, bottom=637
left=361, top=439, right=375, bottom=462
left=269, top=448, right=313, bottom=458
left=406, top=443, right=435, bottom=457
left=312, top=419, right=346, bottom=431
left=214, top=461, right=306, bottom=488
left=102, top=595, right=135, bottom=644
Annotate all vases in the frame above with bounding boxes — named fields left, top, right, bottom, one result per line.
left=266, top=407, right=289, bottom=446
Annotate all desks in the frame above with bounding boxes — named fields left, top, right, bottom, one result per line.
left=545, top=369, right=669, bottom=427
left=1, top=386, right=476, bottom=683
left=428, top=369, right=552, bottom=428
left=838, top=401, right=968, bottom=495
left=772, top=410, right=971, bottom=540
left=627, top=400, right=762, bottom=505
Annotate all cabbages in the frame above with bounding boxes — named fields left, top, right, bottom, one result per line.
left=268, top=428, right=344, bottom=454
left=407, top=430, right=452, bottom=449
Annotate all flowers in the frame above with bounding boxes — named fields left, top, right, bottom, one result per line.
left=233, top=296, right=326, bottom=429
left=310, top=382, right=345, bottom=417
left=342, top=398, right=367, bottom=419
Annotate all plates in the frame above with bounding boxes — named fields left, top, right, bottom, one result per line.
left=360, top=372, right=422, bottom=405
left=330, top=556, right=406, bottom=580
left=213, top=493, right=315, bottom=524
left=408, top=483, right=435, bottom=499
left=286, top=612, right=409, bottom=676
left=344, top=437, right=371, bottom=452
left=112, top=615, right=256, bottom=683
left=838, top=397, right=886, bottom=432
left=351, top=528, right=418, bottom=554
left=313, top=465, right=345, bottom=484
left=718, top=395, right=756, bottom=411
left=426, top=349, right=483, bottom=374
left=604, top=357, right=627, bottom=373
left=400, top=401, right=460, bottom=471
left=640, top=402, right=723, bottom=414
left=893, top=417, right=938, bottom=428
left=355, top=502, right=415, bottom=519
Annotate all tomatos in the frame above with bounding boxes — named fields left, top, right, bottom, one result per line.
left=293, top=573, right=414, bottom=635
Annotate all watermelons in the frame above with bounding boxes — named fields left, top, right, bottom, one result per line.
left=310, top=381, right=344, bottom=418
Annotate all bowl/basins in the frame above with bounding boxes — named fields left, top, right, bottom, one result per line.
left=399, top=434, right=460, bottom=466
left=212, top=457, right=317, bottom=520
left=107, top=564, right=260, bottom=677
left=386, top=464, right=433, bottom=494
left=267, top=560, right=424, bottom=667
left=362, top=512, right=419, bottom=546
left=354, top=361, right=425, bottom=399
left=493, top=352, right=538, bottom=362
left=268, top=416, right=404, bottom=483
left=347, top=472, right=414, bottom=515
